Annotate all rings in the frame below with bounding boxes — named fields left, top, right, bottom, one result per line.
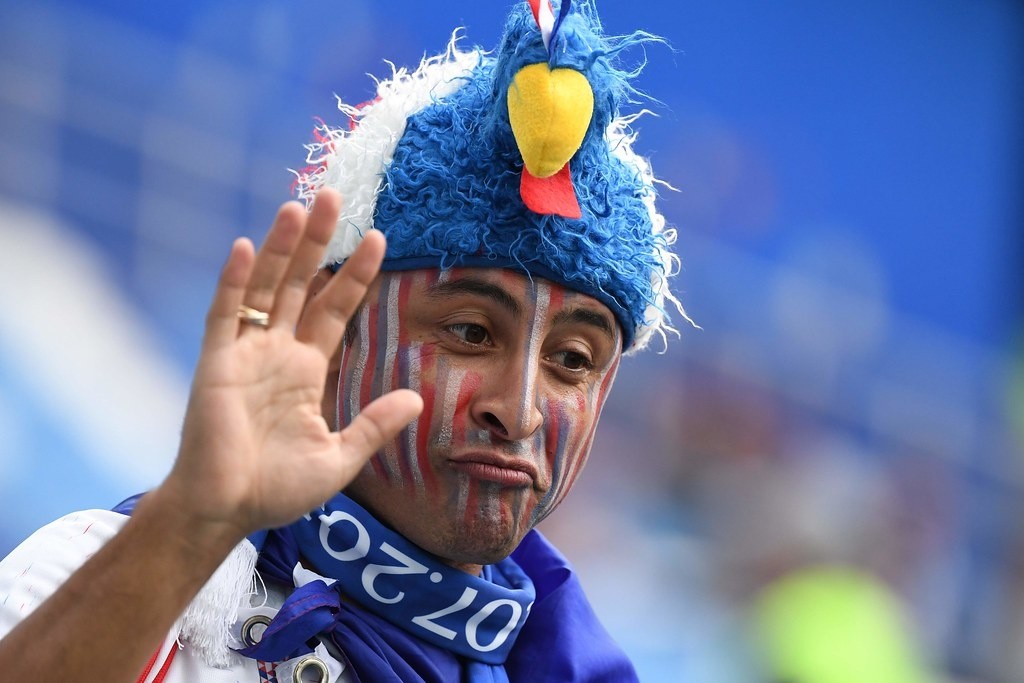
left=238, top=305, right=269, bottom=326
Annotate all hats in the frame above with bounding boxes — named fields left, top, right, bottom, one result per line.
left=287, top=0, right=702, bottom=356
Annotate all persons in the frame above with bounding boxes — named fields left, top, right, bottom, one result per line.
left=0, top=0, right=703, bottom=683
